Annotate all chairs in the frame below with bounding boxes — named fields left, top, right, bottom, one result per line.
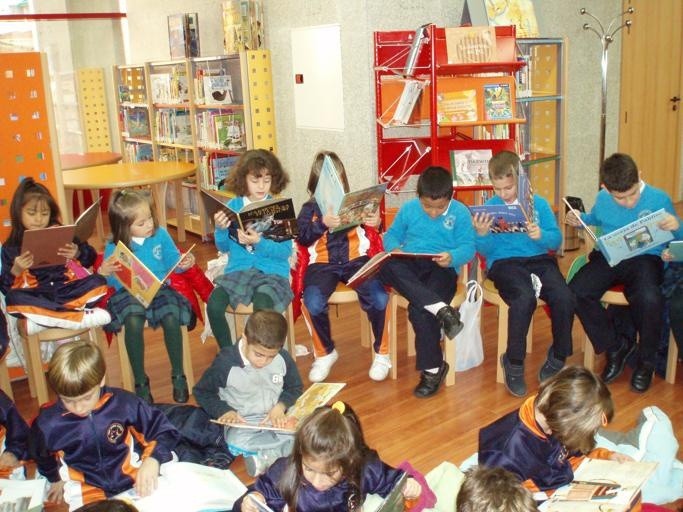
left=228, top=299, right=297, bottom=364
left=582, top=283, right=677, bottom=386
left=476, top=257, right=547, bottom=384
left=1, top=345, right=15, bottom=404
left=113, top=318, right=195, bottom=398
left=388, top=260, right=468, bottom=388
left=20, top=320, right=97, bottom=409
left=329, top=283, right=372, bottom=349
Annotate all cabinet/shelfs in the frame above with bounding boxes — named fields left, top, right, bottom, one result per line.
left=113, top=49, right=277, bottom=242
left=373, top=30, right=565, bottom=255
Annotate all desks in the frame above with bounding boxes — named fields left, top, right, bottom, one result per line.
left=60, top=150, right=207, bottom=242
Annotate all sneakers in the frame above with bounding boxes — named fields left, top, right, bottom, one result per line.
left=435, top=306, right=464, bottom=339
left=602, top=335, right=638, bottom=384
left=369, top=355, right=392, bottom=382
left=501, top=352, right=528, bottom=397
left=309, top=349, right=338, bottom=382
left=540, top=347, right=566, bottom=383
left=415, top=361, right=449, bottom=397
left=631, top=357, right=653, bottom=392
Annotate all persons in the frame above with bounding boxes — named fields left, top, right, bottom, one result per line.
left=0, top=149, right=683, bottom=403
left=0, top=309, right=683, bottom=510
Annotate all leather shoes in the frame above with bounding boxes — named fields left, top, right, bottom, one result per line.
left=136, top=376, right=153, bottom=407
left=172, top=374, right=188, bottom=404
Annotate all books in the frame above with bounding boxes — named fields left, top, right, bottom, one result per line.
left=116, top=0, right=266, bottom=214
left=393, top=25, right=536, bottom=210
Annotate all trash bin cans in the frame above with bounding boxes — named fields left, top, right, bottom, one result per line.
left=565, top=196, right=585, bottom=250
